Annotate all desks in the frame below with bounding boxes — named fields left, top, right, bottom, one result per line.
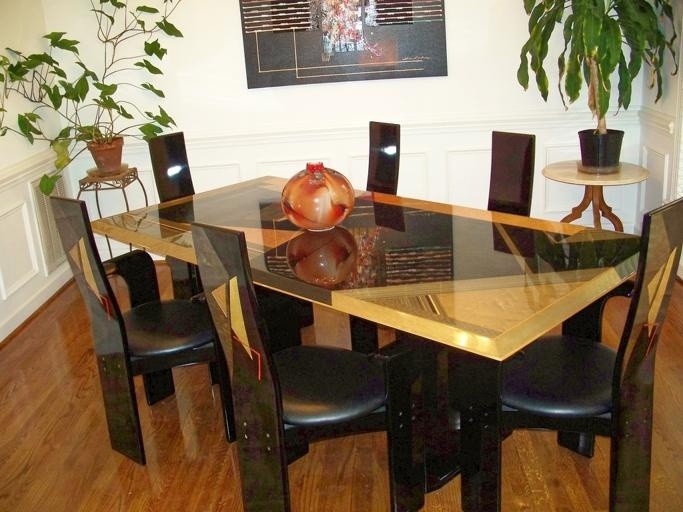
left=75, top=166, right=150, bottom=261
left=541, top=158, right=652, bottom=237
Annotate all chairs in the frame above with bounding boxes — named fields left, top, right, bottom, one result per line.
left=51, top=195, right=236, bottom=468
left=497, top=195, right=683, bottom=512
left=148, top=131, right=200, bottom=201
left=366, top=119, right=402, bottom=196
left=190, top=221, right=389, bottom=512
left=487, top=129, right=537, bottom=216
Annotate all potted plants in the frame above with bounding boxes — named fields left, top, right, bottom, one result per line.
left=0, top=0, right=184, bottom=177
left=517, top=0, right=678, bottom=174
